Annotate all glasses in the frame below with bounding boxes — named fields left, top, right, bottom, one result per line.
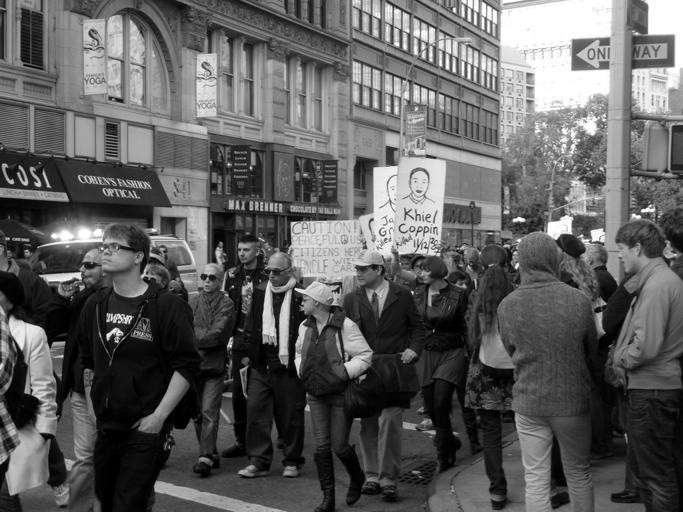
left=201, top=274, right=217, bottom=281
left=264, top=266, right=289, bottom=276
left=143, top=276, right=156, bottom=282
left=78, top=261, right=101, bottom=269
left=99, top=243, right=136, bottom=253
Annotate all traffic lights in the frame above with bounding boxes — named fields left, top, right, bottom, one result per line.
left=667, top=119, right=683, bottom=173
left=640, top=120, right=667, bottom=175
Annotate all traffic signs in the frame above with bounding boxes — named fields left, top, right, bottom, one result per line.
left=572, top=35, right=674, bottom=70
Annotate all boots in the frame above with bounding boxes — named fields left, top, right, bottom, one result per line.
left=312, top=449, right=336, bottom=511
left=337, top=445, right=365, bottom=505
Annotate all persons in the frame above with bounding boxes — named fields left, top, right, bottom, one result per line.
left=141, top=245, right=188, bottom=302
left=384, top=234, right=683, bottom=509
left=343, top=249, right=426, bottom=500
left=1, top=231, right=114, bottom=510
left=1, top=270, right=58, bottom=512
left=498, top=231, right=604, bottom=512
left=611, top=219, right=683, bottom=512
left=402, top=166, right=434, bottom=206
left=190, top=236, right=272, bottom=475
left=237, top=252, right=306, bottom=478
left=294, top=281, right=373, bottom=511
left=80, top=224, right=196, bottom=512
left=378, top=174, right=397, bottom=213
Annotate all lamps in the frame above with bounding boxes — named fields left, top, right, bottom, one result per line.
left=7, top=148, right=30, bottom=177
left=35, top=149, right=54, bottom=174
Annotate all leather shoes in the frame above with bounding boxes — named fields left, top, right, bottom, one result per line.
left=610, top=490, right=644, bottom=503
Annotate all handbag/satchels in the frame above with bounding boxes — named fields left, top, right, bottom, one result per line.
left=346, top=353, right=420, bottom=419
left=477, top=311, right=514, bottom=369
left=201, top=349, right=227, bottom=376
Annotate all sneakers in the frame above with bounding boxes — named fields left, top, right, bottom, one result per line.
left=52, top=484, right=70, bottom=507
left=416, top=418, right=434, bottom=431
left=193, top=452, right=219, bottom=474
left=492, top=497, right=510, bottom=508
left=380, top=485, right=398, bottom=500
left=237, top=465, right=271, bottom=477
left=222, top=444, right=246, bottom=458
left=283, top=464, right=302, bottom=477
left=552, top=491, right=570, bottom=509
left=363, top=480, right=380, bottom=494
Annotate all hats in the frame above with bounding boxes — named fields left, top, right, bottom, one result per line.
left=558, top=234, right=586, bottom=258
left=293, top=280, right=334, bottom=307
left=349, top=250, right=384, bottom=266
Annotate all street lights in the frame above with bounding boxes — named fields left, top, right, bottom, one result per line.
left=399, top=36, right=472, bottom=165
left=468, top=200, right=475, bottom=247
left=547, top=154, right=603, bottom=224
left=511, top=216, right=526, bottom=233
left=640, top=207, right=654, bottom=219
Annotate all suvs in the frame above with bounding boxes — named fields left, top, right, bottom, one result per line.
left=21, top=228, right=198, bottom=339
